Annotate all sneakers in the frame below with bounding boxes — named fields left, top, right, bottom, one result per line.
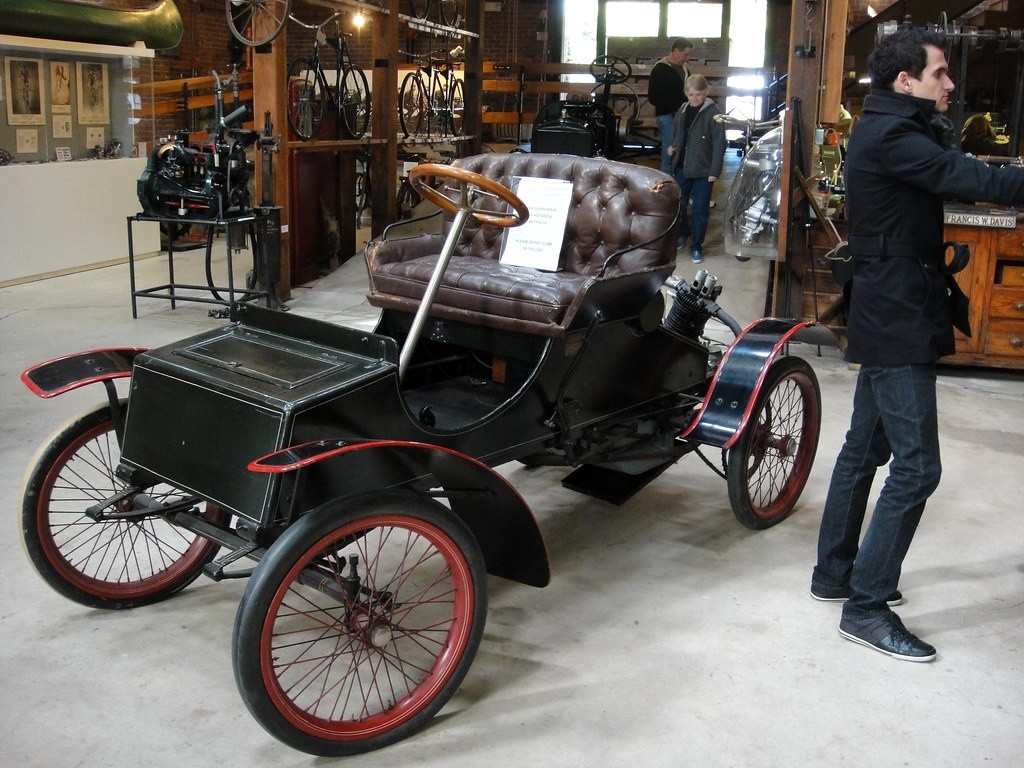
left=838, top=611, right=937, bottom=662
left=810, top=575, right=902, bottom=606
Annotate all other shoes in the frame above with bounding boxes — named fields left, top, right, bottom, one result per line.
left=692, top=250, right=703, bottom=263
left=676, top=236, right=688, bottom=250
left=689, top=198, right=716, bottom=208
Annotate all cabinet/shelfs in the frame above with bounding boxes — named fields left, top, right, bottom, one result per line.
left=941, top=203, right=991, bottom=365
left=787, top=203, right=848, bottom=344
left=983, top=207, right=1024, bottom=369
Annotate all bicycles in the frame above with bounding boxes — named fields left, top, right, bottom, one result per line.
left=287, top=11, right=370, bottom=140
left=399, top=48, right=464, bottom=139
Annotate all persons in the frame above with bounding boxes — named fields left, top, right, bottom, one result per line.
left=809, top=29, right=1023, bottom=662
left=668, top=74, right=725, bottom=264
left=647, top=39, right=718, bottom=207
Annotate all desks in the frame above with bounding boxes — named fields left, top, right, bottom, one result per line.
left=126, top=212, right=273, bottom=324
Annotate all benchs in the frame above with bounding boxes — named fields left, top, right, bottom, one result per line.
left=362, top=152, right=683, bottom=338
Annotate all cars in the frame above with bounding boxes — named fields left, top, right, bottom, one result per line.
left=510, top=55, right=663, bottom=172
left=21, top=152, right=823, bottom=758
